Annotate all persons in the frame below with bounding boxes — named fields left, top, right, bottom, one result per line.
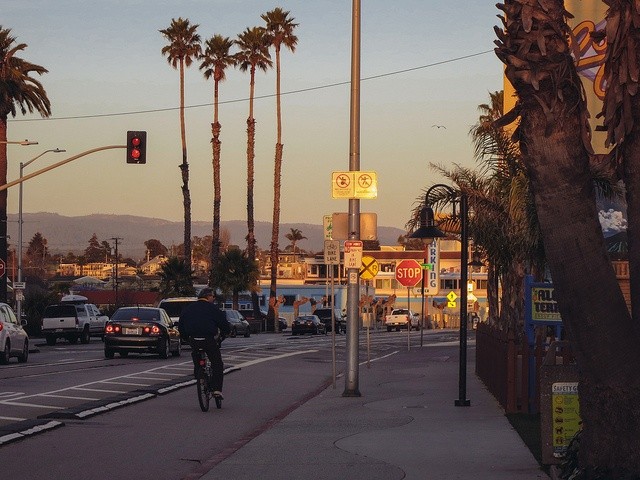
left=178, top=288, right=232, bottom=400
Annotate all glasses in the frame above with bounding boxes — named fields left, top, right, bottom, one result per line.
left=210, top=294, right=215, bottom=298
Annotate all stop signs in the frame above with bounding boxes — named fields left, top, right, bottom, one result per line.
left=358, top=258, right=379, bottom=279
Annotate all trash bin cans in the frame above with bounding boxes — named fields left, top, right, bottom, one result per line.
left=540, top=340, right=578, bottom=480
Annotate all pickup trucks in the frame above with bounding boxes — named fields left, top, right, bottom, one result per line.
left=384, top=308, right=420, bottom=332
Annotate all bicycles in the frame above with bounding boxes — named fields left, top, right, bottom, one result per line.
left=180, top=337, right=225, bottom=413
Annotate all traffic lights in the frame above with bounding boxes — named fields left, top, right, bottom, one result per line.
left=127, top=129, right=145, bottom=166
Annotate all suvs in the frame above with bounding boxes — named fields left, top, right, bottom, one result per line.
left=221, top=309, right=250, bottom=338
left=157, top=296, right=199, bottom=331
left=39, top=295, right=110, bottom=346
left=238, top=309, right=287, bottom=331
left=0, top=301, right=30, bottom=366
left=312, top=308, right=348, bottom=334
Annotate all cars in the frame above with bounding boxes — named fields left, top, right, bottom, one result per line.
left=291, top=314, right=327, bottom=336
left=103, top=305, right=182, bottom=359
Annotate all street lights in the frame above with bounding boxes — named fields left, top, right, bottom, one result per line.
left=411, top=183, right=473, bottom=407
left=17, top=148, right=67, bottom=323
left=0, top=138, right=36, bottom=151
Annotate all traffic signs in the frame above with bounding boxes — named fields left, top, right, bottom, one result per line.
left=444, top=287, right=458, bottom=309
left=332, top=172, right=376, bottom=200
left=324, top=239, right=340, bottom=264
left=466, top=284, right=477, bottom=309
left=395, top=259, right=423, bottom=288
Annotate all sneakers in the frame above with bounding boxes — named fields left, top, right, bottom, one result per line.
left=214, top=391, right=224, bottom=400
